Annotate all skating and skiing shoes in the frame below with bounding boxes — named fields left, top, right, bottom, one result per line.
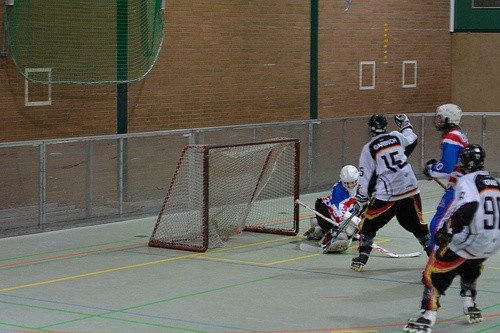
left=462, top=306, right=484, bottom=324
left=402, top=311, right=437, bottom=333
left=349, top=253, right=368, bottom=272
left=302, top=226, right=324, bottom=240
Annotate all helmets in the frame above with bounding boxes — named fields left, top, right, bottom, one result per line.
left=457, top=143, right=487, bottom=171
left=338, top=165, right=361, bottom=183
left=367, top=114, right=390, bottom=132
left=434, top=104, right=463, bottom=129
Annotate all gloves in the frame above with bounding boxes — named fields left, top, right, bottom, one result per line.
left=351, top=199, right=368, bottom=216
left=425, top=231, right=450, bottom=258
left=423, top=159, right=437, bottom=180
left=340, top=213, right=361, bottom=238
left=393, top=114, right=409, bottom=128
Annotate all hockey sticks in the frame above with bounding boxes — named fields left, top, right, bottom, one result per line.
left=294, top=199, right=421, bottom=258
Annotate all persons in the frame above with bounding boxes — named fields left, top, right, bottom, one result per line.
left=423, top=104, right=471, bottom=251
left=402, top=143, right=500, bottom=333
left=302, top=164, right=368, bottom=253
left=350, top=113, right=434, bottom=272
